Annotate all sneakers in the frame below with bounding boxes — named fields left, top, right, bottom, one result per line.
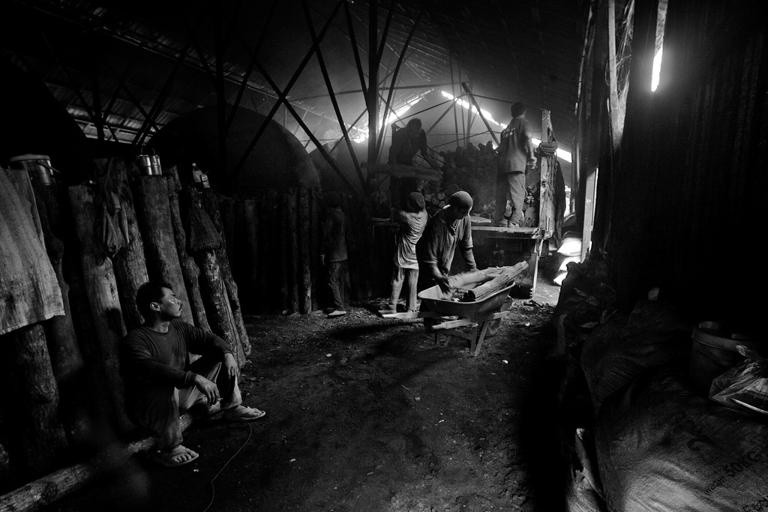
left=328, top=310, right=347, bottom=316
left=378, top=309, right=396, bottom=314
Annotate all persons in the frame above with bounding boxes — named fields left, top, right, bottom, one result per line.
left=489, top=102, right=535, bottom=227
left=415, top=189, right=478, bottom=333
left=386, top=118, right=444, bottom=210
left=119, top=280, right=266, bottom=468
left=320, top=191, right=350, bottom=318
left=377, top=191, right=429, bottom=315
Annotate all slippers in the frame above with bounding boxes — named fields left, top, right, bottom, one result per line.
left=157, top=445, right=199, bottom=465
left=234, top=405, right=266, bottom=420
